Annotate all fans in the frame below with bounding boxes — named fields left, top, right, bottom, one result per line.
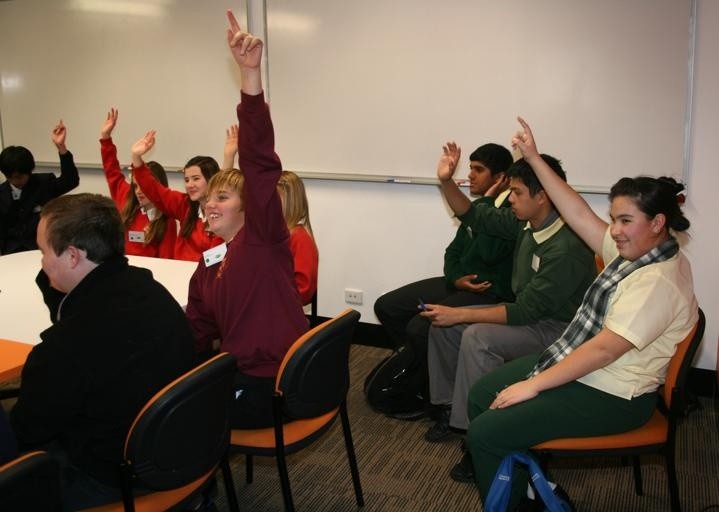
left=0, top=0, right=249, bottom=174
left=262, top=0, right=698, bottom=198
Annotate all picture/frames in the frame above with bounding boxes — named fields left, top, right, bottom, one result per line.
left=1, top=247, right=202, bottom=408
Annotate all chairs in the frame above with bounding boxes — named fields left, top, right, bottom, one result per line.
left=449, top=452, right=479, bottom=484
left=183, top=498, right=219, bottom=512
left=424, top=410, right=468, bottom=443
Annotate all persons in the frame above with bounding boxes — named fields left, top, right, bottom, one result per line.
left=416, top=140, right=601, bottom=483
left=131, top=129, right=225, bottom=261
left=2, top=118, right=80, bottom=255
left=373, top=141, right=518, bottom=421
left=182, top=6, right=317, bottom=434
left=0, top=191, right=213, bottom=510
left=98, top=107, right=177, bottom=259
left=462, top=112, right=702, bottom=510
left=222, top=124, right=318, bottom=305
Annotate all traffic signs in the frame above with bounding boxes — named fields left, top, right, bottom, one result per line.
left=343, top=287, right=365, bottom=305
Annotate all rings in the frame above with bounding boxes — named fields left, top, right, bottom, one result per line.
left=455, top=182, right=471, bottom=186
left=417, top=297, right=431, bottom=320
left=387, top=179, right=412, bottom=183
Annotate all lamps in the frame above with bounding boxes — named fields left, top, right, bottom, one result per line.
left=0, top=449, right=64, bottom=510
left=524, top=304, right=707, bottom=508
left=301, top=291, right=318, bottom=331
left=200, top=308, right=368, bottom=509
left=78, top=349, right=242, bottom=511
